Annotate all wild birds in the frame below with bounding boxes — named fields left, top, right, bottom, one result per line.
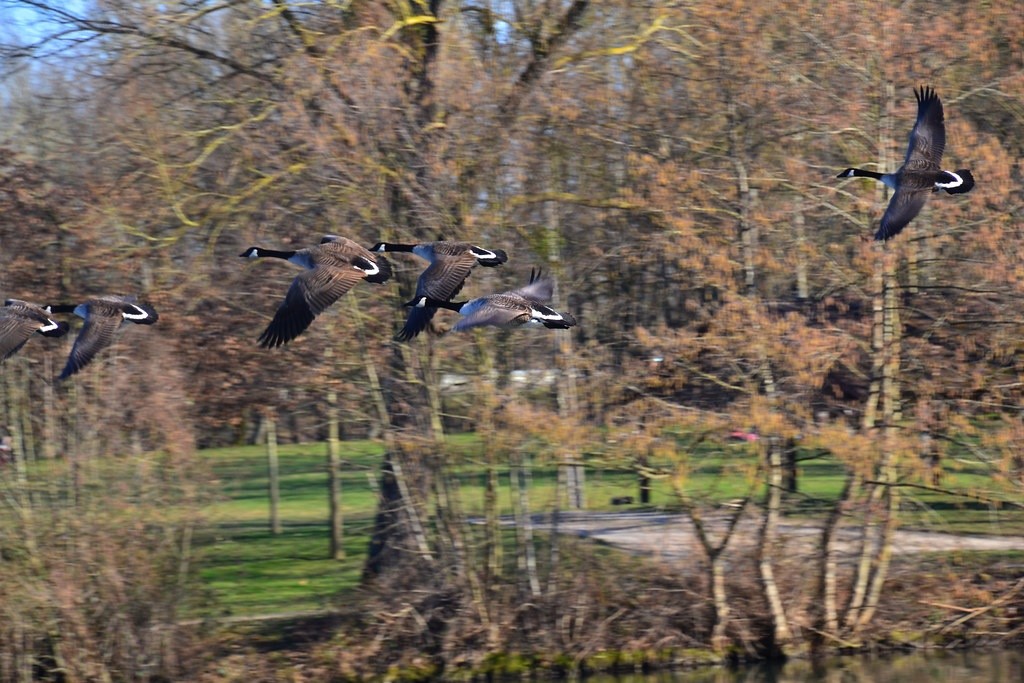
left=367, top=241, right=508, bottom=343
left=837, top=84, right=975, bottom=244
left=0, top=299, right=70, bottom=362
left=41, top=293, right=158, bottom=379
left=238, top=235, right=392, bottom=350
left=400, top=265, right=576, bottom=335
